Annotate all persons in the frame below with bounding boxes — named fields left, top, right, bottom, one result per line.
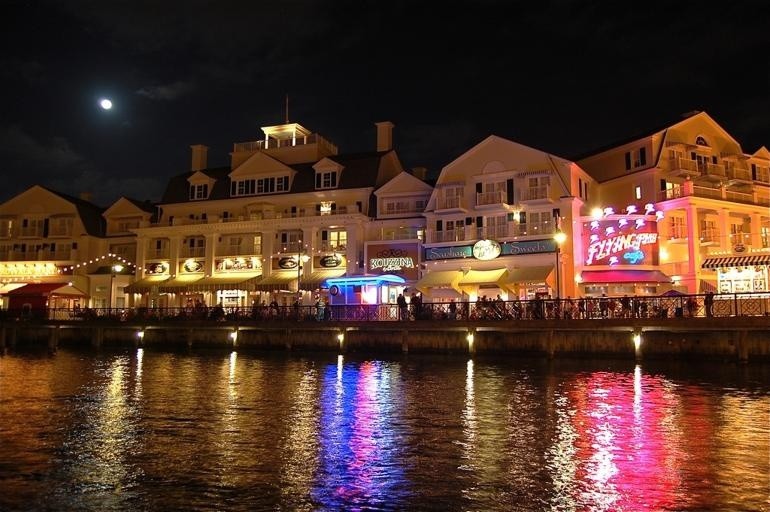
left=73, top=298, right=225, bottom=321
left=397, top=293, right=561, bottom=322
left=227, top=299, right=332, bottom=321
left=563, top=292, right=715, bottom=321
